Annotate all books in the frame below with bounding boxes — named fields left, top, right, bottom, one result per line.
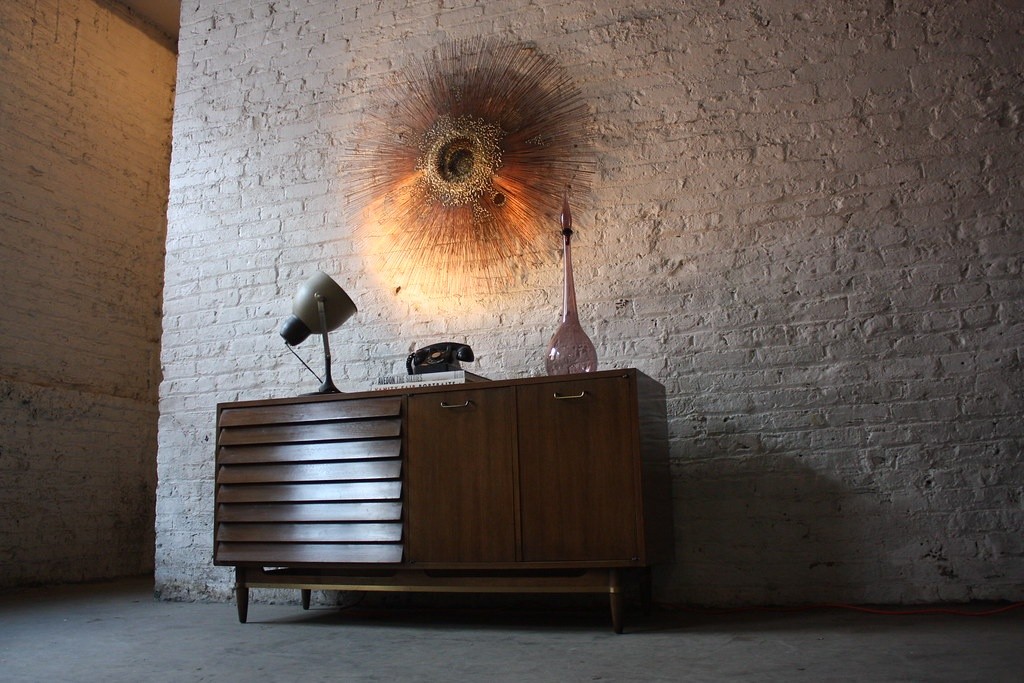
left=370, top=369, right=493, bottom=392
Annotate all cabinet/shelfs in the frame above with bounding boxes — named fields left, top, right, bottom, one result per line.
left=215, top=367, right=677, bottom=631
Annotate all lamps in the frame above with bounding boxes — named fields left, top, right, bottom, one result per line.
left=279, top=271, right=357, bottom=397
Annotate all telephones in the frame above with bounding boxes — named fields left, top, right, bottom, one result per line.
left=406, top=342, right=475, bottom=375
